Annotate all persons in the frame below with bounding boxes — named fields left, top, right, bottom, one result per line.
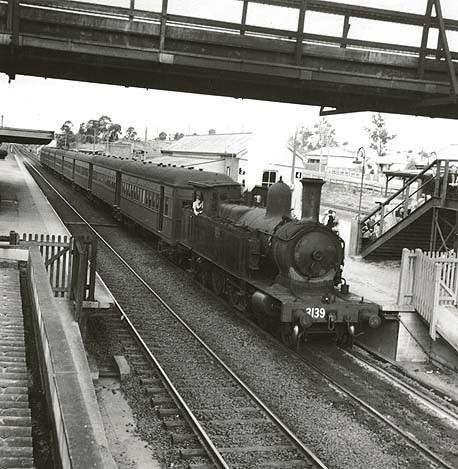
left=325, top=210, right=334, bottom=230
left=322, top=211, right=328, bottom=224
left=290, top=208, right=297, bottom=220
left=332, top=211, right=339, bottom=230
left=192, top=195, right=204, bottom=216
left=361, top=193, right=431, bottom=242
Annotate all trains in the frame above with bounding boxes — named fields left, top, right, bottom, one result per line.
left=40, top=146, right=382, bottom=353
